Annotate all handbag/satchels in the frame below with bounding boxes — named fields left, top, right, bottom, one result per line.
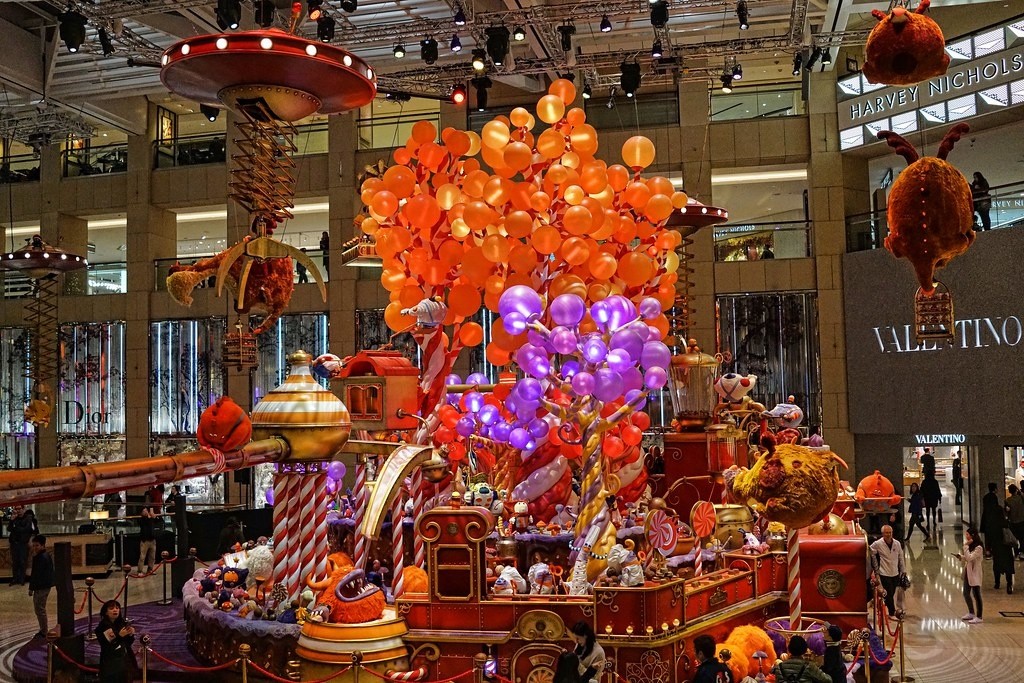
left=1003, top=527, right=1018, bottom=547
left=938, top=503, right=942, bottom=522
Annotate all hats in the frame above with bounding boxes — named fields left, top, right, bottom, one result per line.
left=824, top=621, right=842, bottom=642
left=789, top=635, right=808, bottom=655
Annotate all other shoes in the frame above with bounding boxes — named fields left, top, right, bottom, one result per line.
left=986, top=553, right=992, bottom=560
left=962, top=614, right=975, bottom=619
left=33, top=632, right=47, bottom=639
left=137, top=571, right=142, bottom=577
left=969, top=617, right=983, bottom=623
left=995, top=584, right=1000, bottom=589
left=9, top=580, right=18, bottom=586
left=923, top=535, right=930, bottom=541
left=1008, top=587, right=1012, bottom=594
left=21, top=580, right=25, bottom=586
left=146, top=569, right=157, bottom=575
left=904, top=538, right=910, bottom=541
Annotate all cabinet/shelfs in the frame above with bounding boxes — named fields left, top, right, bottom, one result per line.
left=221, top=338, right=259, bottom=367
left=915, top=281, right=955, bottom=346
left=918, top=458, right=954, bottom=480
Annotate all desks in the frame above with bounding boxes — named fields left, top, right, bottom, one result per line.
left=116, top=530, right=175, bottom=566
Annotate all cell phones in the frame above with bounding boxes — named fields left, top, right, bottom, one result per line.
left=125, top=621, right=131, bottom=630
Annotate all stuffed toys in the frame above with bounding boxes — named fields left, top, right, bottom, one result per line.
left=197, top=396, right=252, bottom=452
left=307, top=558, right=386, bottom=623
left=714, top=625, right=777, bottom=683
left=862, top=0, right=950, bottom=86
left=876, top=122, right=976, bottom=295
left=854, top=470, right=901, bottom=512
left=202, top=541, right=288, bottom=620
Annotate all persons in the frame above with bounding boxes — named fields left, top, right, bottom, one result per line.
left=978, top=480, right=1024, bottom=594
left=819, top=626, right=847, bottom=683
left=6, top=506, right=32, bottom=586
left=148, top=486, right=163, bottom=519
left=94, top=599, right=141, bottom=683
left=104, top=493, right=118, bottom=535
left=320, top=231, right=329, bottom=271
left=904, top=483, right=930, bottom=542
left=921, top=448, right=942, bottom=528
left=136, top=506, right=157, bottom=575
left=692, top=634, right=734, bottom=683
left=956, top=528, right=986, bottom=623
left=953, top=458, right=961, bottom=505
left=28, top=535, right=54, bottom=639
left=971, top=171, right=991, bottom=231
left=165, top=485, right=181, bottom=533
left=869, top=525, right=907, bottom=621
left=296, top=248, right=308, bottom=283
left=552, top=622, right=605, bottom=683
left=771, top=635, right=832, bottom=683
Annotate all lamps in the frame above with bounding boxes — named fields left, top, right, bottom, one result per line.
left=234, top=319, right=243, bottom=331
left=59, top=0, right=832, bottom=122
left=90, top=511, right=109, bottom=534
left=932, top=276, right=938, bottom=287
